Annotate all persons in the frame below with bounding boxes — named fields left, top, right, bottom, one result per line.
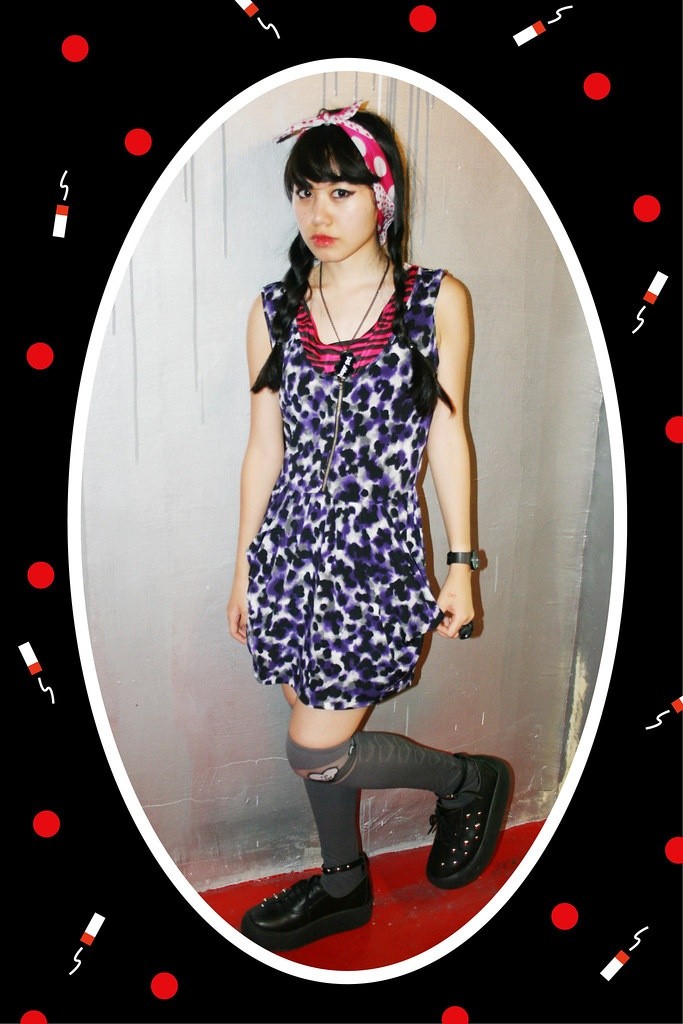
left=225, top=98, right=510, bottom=952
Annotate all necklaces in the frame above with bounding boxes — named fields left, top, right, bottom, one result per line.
left=319, top=254, right=390, bottom=377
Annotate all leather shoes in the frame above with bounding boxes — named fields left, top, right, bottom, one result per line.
left=241, top=853, right=372, bottom=950
left=426, top=751, right=511, bottom=888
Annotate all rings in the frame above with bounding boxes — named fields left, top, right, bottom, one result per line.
left=459, top=622, right=473, bottom=639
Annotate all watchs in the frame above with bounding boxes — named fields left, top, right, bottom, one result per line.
left=447, top=550, right=480, bottom=570
